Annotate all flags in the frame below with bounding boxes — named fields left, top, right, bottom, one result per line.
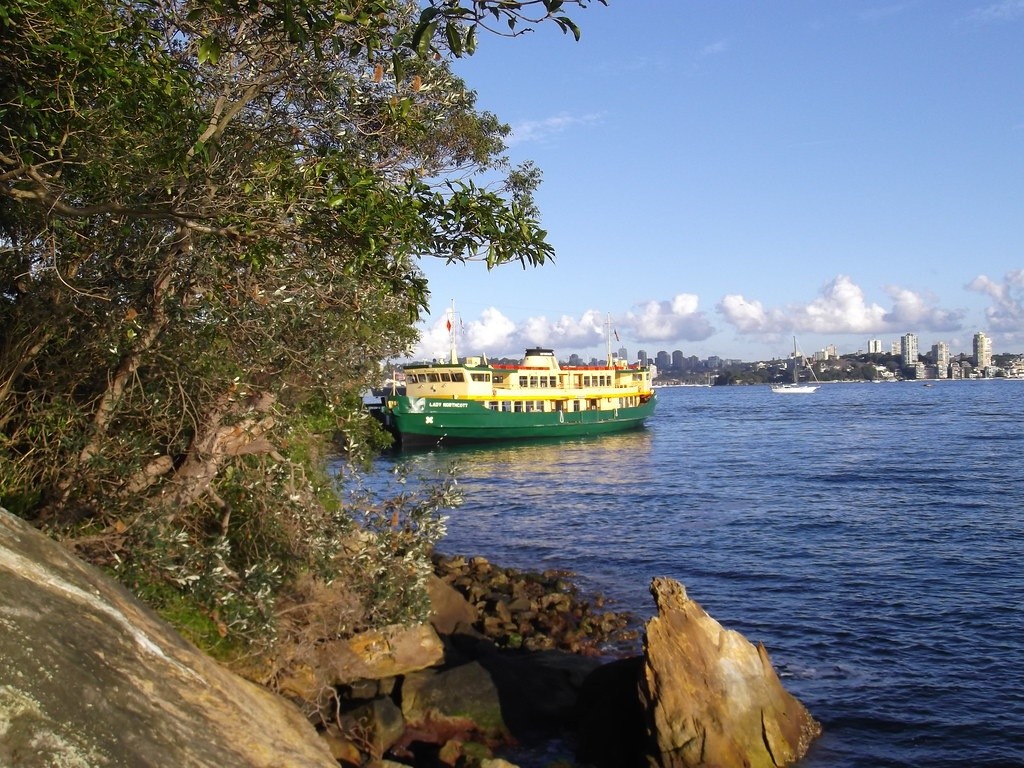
left=446, top=319, right=451, bottom=331
left=614, top=328, right=619, bottom=341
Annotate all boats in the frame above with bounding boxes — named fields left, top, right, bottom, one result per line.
left=770, top=335, right=819, bottom=394
left=362, top=296, right=657, bottom=445
